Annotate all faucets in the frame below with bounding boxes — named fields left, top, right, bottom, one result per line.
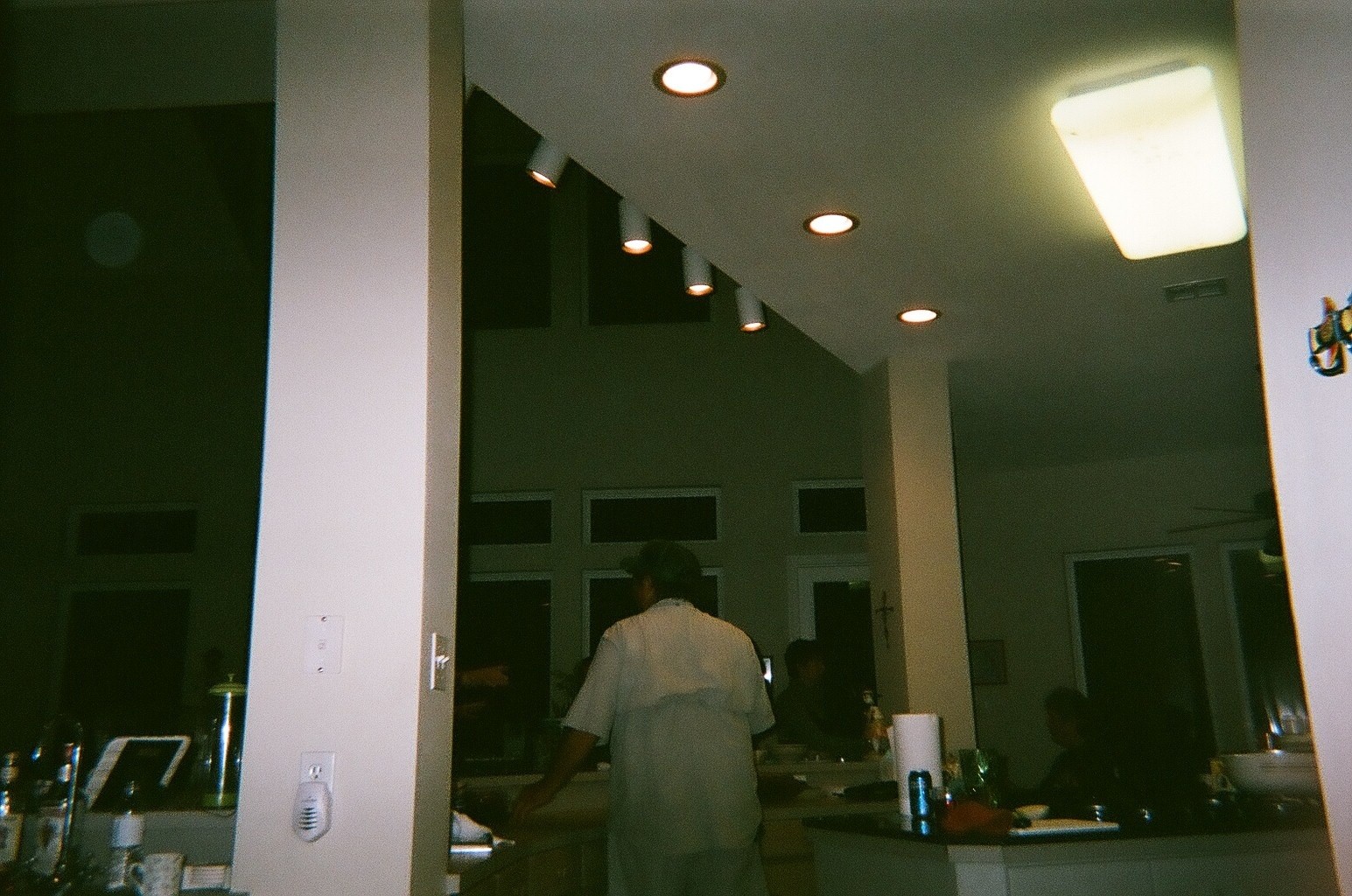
left=31, top=715, right=95, bottom=883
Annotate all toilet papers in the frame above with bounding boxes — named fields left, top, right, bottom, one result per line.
left=885, top=713, right=945, bottom=817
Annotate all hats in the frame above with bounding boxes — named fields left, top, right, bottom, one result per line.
left=620, top=540, right=703, bottom=601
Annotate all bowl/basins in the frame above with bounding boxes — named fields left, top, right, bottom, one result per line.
left=1215, top=752, right=1321, bottom=800
left=1016, top=804, right=1049, bottom=822
left=463, top=757, right=516, bottom=776
left=752, top=749, right=769, bottom=766
left=773, top=743, right=808, bottom=764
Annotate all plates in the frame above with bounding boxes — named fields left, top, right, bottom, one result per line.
left=844, top=781, right=899, bottom=801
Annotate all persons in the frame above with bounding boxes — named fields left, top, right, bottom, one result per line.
left=454, top=664, right=510, bottom=720
left=1035, top=686, right=1093, bottom=810
left=748, top=635, right=833, bottom=743
left=515, top=541, right=776, bottom=896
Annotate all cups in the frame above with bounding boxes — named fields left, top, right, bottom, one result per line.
left=126, top=851, right=184, bottom=896
left=957, top=747, right=1000, bottom=809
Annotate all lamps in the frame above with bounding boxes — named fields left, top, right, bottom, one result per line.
left=527, top=133, right=766, bottom=332
left=1050, top=57, right=1247, bottom=261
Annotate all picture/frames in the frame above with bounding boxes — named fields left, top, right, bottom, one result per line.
left=967, top=640, right=1007, bottom=685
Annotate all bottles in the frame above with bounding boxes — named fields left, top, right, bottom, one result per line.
left=0, top=733, right=74, bottom=896
left=106, top=780, right=145, bottom=893
left=199, top=672, right=247, bottom=808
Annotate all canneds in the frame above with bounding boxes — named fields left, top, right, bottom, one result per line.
left=0, top=752, right=22, bottom=784
left=908, top=769, right=934, bottom=817
